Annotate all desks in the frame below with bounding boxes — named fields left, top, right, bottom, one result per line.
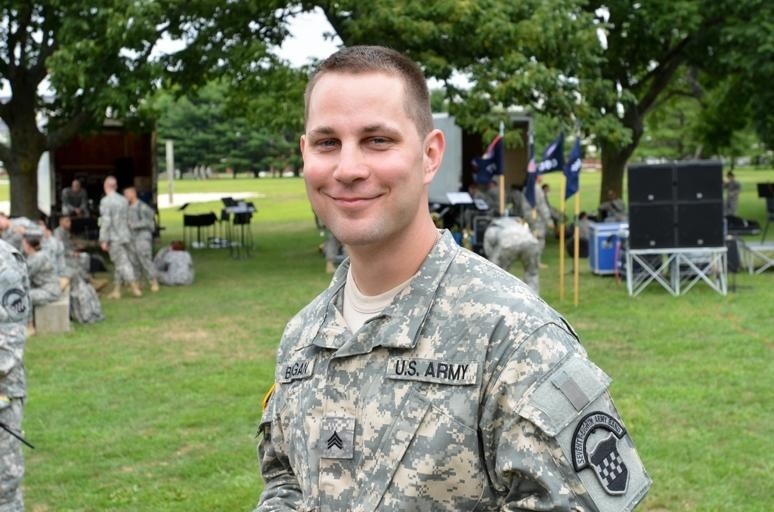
left=738, top=238, right=774, bottom=276
left=622, top=244, right=732, bottom=299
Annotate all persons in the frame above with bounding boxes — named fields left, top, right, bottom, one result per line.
left=325, top=230, right=350, bottom=274
left=722, top=171, right=741, bottom=215
left=0, top=175, right=196, bottom=337
left=0, top=240, right=30, bottom=512
left=256, top=45, right=654, bottom=512
left=464, top=182, right=628, bottom=295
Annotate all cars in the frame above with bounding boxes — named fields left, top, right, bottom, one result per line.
left=642, top=154, right=766, bottom=168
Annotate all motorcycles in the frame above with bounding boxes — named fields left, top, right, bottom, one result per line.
left=567, top=193, right=627, bottom=258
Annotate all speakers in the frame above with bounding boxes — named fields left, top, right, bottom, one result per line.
left=626, top=162, right=740, bottom=281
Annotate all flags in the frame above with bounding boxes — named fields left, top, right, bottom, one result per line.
left=564, top=138, right=582, bottom=202
left=536, top=133, right=564, bottom=175
left=521, top=136, right=537, bottom=209
left=470, top=119, right=504, bottom=186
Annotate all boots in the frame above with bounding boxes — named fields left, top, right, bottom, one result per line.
left=130, top=281, right=143, bottom=298
left=149, top=276, right=160, bottom=293
left=106, top=286, right=122, bottom=300
left=89, top=277, right=110, bottom=292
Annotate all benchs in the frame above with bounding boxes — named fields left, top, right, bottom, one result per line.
left=30, top=277, right=72, bottom=336
